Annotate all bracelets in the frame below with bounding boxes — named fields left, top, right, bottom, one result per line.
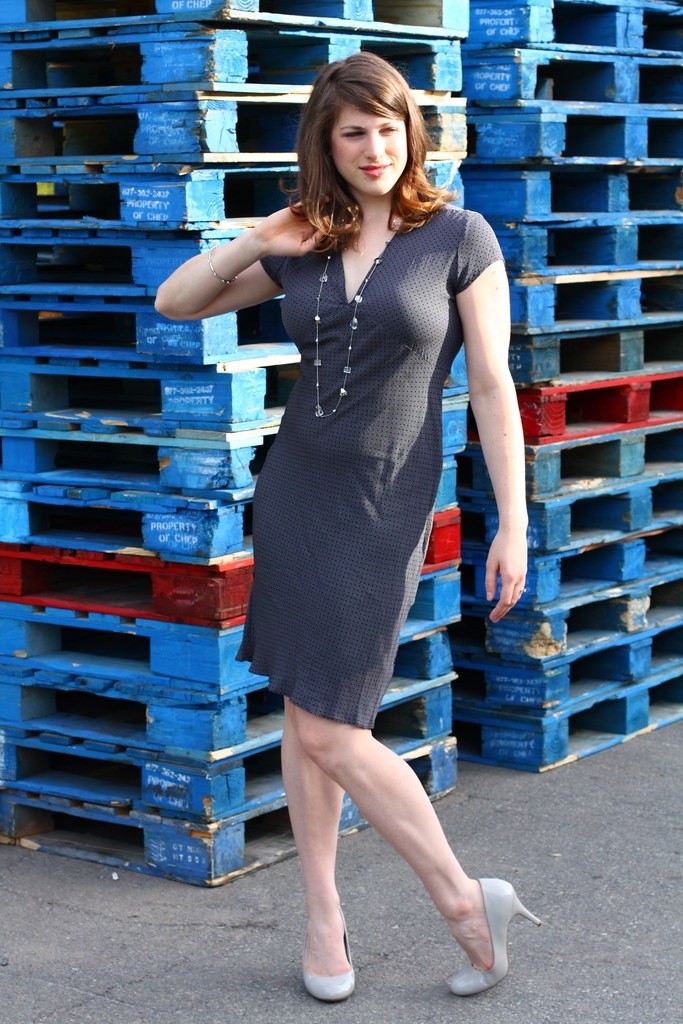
left=208, top=246, right=237, bottom=284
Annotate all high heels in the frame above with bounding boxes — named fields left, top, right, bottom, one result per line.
left=450, top=878, right=542, bottom=997
left=303, top=905, right=355, bottom=1000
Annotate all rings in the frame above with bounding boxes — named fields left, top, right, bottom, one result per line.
left=521, top=588, right=526, bottom=592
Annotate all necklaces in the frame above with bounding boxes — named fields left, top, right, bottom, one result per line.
left=314, top=220, right=400, bottom=419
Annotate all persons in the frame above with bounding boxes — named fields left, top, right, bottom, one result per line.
left=156, top=53, right=542, bottom=999
